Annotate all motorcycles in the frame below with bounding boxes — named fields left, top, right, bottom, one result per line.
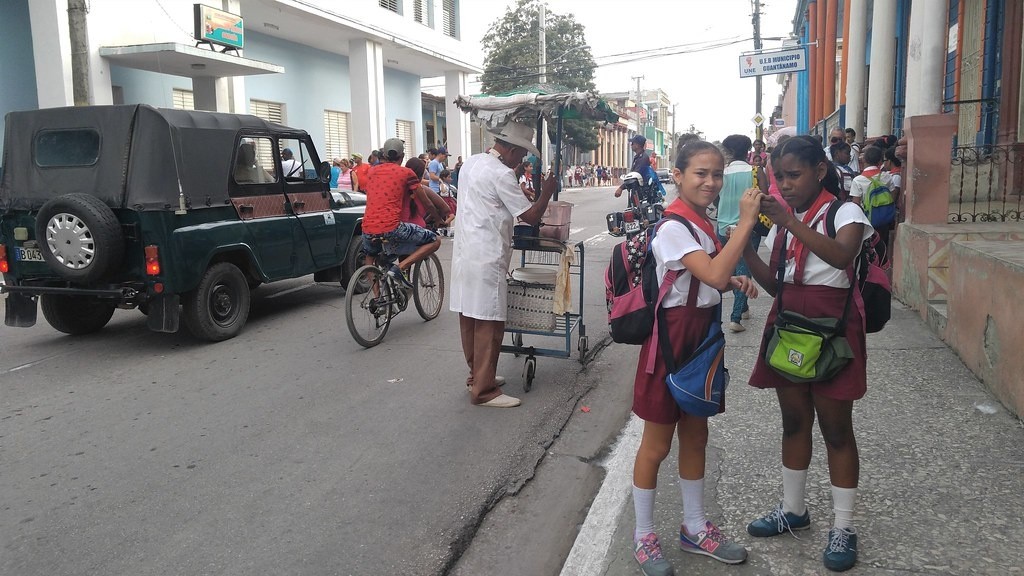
left=605, top=178, right=669, bottom=240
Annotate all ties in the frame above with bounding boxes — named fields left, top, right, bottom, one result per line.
left=525, top=173, right=532, bottom=182
left=861, top=169, right=881, bottom=177
left=663, top=195, right=722, bottom=253
left=889, top=167, right=902, bottom=173
left=787, top=187, right=834, bottom=285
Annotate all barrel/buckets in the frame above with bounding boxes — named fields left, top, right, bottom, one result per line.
left=513, top=267, right=557, bottom=286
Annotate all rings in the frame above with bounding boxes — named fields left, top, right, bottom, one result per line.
left=746, top=192, right=753, bottom=196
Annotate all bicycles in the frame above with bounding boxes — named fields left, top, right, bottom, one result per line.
left=345, top=222, right=449, bottom=348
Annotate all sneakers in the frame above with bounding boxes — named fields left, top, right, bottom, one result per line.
left=375, top=298, right=387, bottom=311
left=387, top=265, right=414, bottom=290
left=823, top=521, right=857, bottom=571
left=679, top=519, right=748, bottom=564
left=747, top=501, right=811, bottom=541
left=633, top=525, right=674, bottom=576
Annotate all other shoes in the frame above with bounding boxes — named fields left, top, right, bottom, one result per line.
left=729, top=321, right=746, bottom=331
left=741, top=309, right=749, bottom=319
left=475, top=391, right=521, bottom=407
left=468, top=375, right=505, bottom=392
left=446, top=233, right=454, bottom=238
left=431, top=227, right=444, bottom=237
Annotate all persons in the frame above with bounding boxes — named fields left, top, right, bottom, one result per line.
left=649, top=151, right=657, bottom=171
left=362, top=138, right=441, bottom=314
left=631, top=135, right=765, bottom=576
left=565, top=160, right=614, bottom=188
left=716, top=123, right=909, bottom=332
left=449, top=122, right=561, bottom=409
left=615, top=135, right=650, bottom=208
left=281, top=145, right=563, bottom=288
left=726, top=134, right=875, bottom=572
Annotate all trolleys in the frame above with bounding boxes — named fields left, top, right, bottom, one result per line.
left=500, top=236, right=590, bottom=394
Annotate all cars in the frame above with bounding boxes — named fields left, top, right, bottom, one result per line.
left=654, top=168, right=674, bottom=184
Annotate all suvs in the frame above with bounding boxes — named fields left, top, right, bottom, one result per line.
left=0, top=103, right=368, bottom=343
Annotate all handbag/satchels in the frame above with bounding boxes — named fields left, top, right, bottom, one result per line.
left=761, top=307, right=856, bottom=385
left=503, top=271, right=557, bottom=333
left=666, top=319, right=725, bottom=417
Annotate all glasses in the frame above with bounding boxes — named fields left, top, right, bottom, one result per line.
left=831, top=136, right=846, bottom=143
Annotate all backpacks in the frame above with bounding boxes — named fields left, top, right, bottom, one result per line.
left=861, top=170, right=895, bottom=229
left=777, top=192, right=889, bottom=332
left=603, top=213, right=694, bottom=347
left=752, top=165, right=773, bottom=228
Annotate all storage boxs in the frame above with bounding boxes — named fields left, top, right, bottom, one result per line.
left=607, top=212, right=626, bottom=235
left=645, top=204, right=665, bottom=224
left=516, top=200, right=574, bottom=246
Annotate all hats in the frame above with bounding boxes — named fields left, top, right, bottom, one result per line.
left=484, top=119, right=540, bottom=160
left=352, top=152, right=363, bottom=161
left=628, top=134, right=646, bottom=145
left=437, top=147, right=452, bottom=158
left=383, top=138, right=405, bottom=158
left=332, top=157, right=341, bottom=165
